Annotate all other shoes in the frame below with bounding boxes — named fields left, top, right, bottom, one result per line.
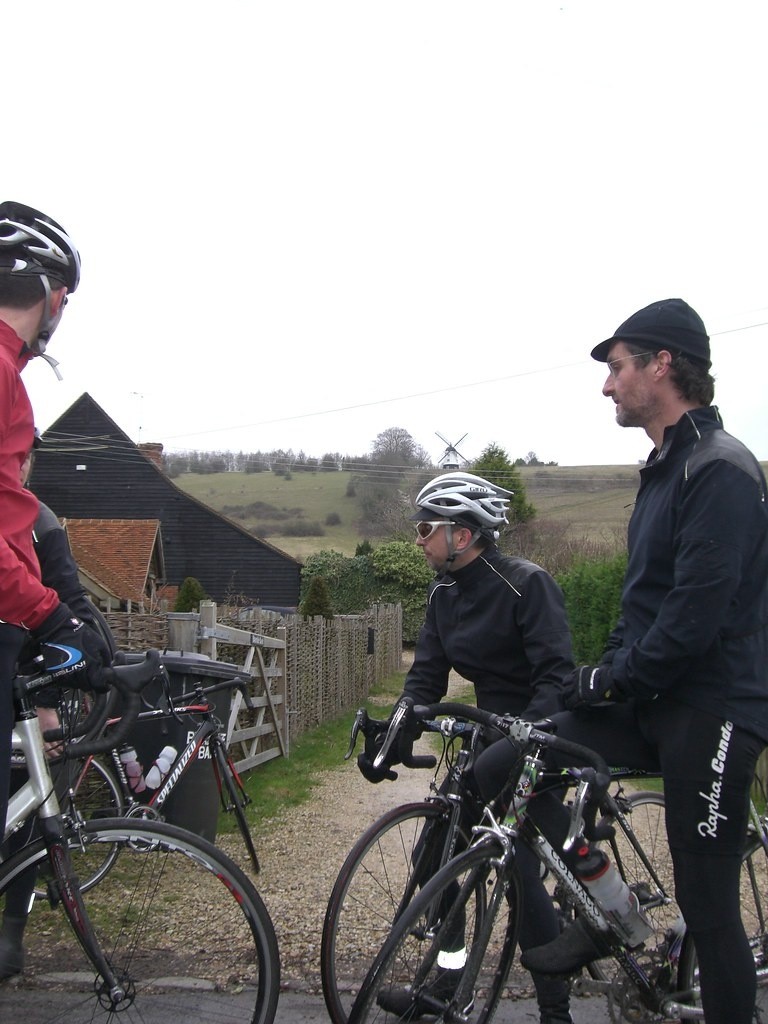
left=0, top=913, right=28, bottom=976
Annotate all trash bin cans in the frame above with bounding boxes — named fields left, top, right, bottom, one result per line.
left=111, top=650, right=245, bottom=853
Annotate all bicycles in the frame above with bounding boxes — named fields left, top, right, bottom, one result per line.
left=317, top=695, right=768, bottom=1024
left=0, top=595, right=280, bottom=1023
left=31, top=675, right=260, bottom=899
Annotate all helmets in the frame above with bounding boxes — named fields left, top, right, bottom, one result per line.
left=414, top=472, right=515, bottom=526
left=0, top=201, right=82, bottom=294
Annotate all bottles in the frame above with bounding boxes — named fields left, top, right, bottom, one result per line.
left=144, top=746, right=178, bottom=789
left=572, top=846, right=653, bottom=947
left=119, top=743, right=146, bottom=793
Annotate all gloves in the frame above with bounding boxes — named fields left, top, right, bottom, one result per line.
left=562, top=664, right=615, bottom=711
left=356, top=731, right=416, bottom=783
left=27, top=608, right=115, bottom=693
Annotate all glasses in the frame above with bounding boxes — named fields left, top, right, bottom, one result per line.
left=415, top=521, right=460, bottom=540
left=606, top=351, right=656, bottom=379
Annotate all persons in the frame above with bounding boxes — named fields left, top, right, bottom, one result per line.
left=473, top=300, right=768, bottom=1024
left=0, top=202, right=111, bottom=990
left=357, top=473, right=575, bottom=1024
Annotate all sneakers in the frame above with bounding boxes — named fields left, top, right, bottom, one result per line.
left=377, top=984, right=474, bottom=1019
left=521, top=916, right=617, bottom=975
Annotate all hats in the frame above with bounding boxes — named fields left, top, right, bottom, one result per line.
left=590, top=298, right=711, bottom=366
left=410, top=508, right=494, bottom=540
